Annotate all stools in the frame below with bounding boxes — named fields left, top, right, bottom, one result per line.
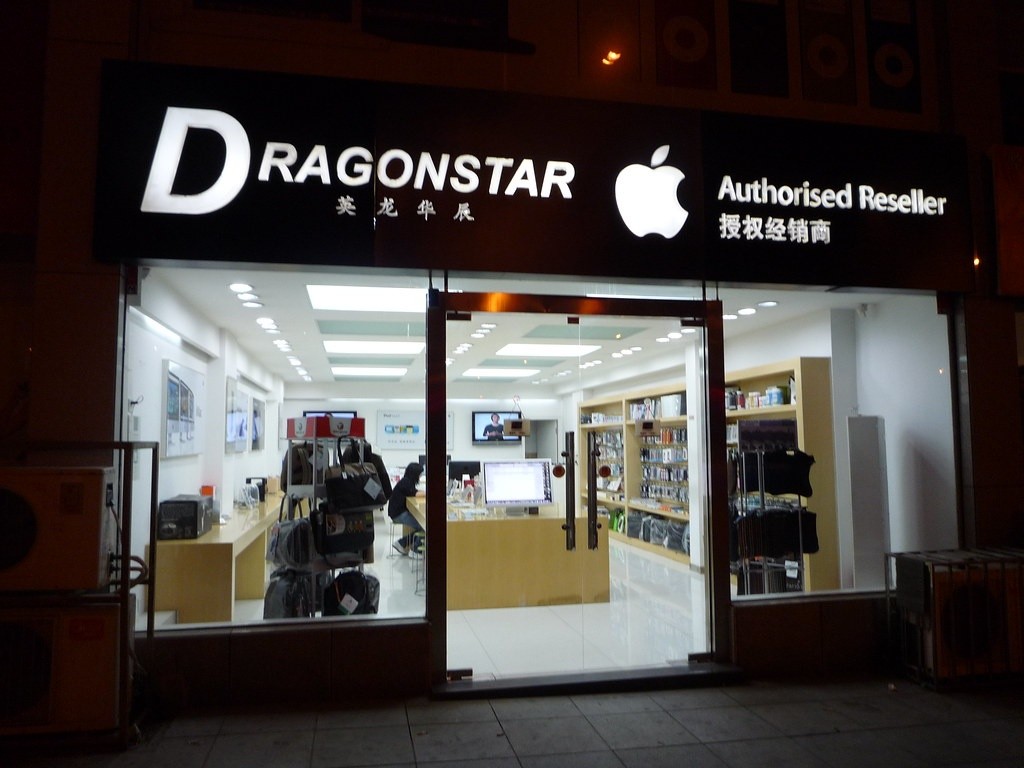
left=388, top=521, right=412, bottom=556
left=410, top=531, right=426, bottom=598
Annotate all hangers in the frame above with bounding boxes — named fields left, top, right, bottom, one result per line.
left=727, top=443, right=809, bottom=457
left=732, top=494, right=797, bottom=511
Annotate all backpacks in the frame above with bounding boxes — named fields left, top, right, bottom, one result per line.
left=280, top=440, right=329, bottom=494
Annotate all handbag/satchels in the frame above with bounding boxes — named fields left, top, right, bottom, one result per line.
left=316, top=572, right=380, bottom=616
left=269, top=494, right=375, bottom=568
left=263, top=568, right=311, bottom=619
left=325, top=434, right=392, bottom=515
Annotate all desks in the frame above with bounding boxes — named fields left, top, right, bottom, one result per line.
left=145, top=485, right=307, bottom=623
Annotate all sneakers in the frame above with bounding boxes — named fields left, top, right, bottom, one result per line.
left=409, top=551, right=422, bottom=560
left=392, top=541, right=408, bottom=555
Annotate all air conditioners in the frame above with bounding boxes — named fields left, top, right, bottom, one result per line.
left=908, top=562, right=1024, bottom=677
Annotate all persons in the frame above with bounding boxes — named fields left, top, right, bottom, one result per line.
left=388, top=463, right=424, bottom=559
left=483, top=412, right=503, bottom=440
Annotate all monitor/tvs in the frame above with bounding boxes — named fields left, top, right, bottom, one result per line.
left=419, top=455, right=553, bottom=517
left=471, top=411, right=522, bottom=445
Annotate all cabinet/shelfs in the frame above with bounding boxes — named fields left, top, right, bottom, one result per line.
left=287, top=415, right=366, bottom=618
left=579, top=354, right=839, bottom=593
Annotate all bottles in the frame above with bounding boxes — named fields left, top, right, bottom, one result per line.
left=729, top=385, right=746, bottom=411
left=610, top=507, right=626, bottom=534
